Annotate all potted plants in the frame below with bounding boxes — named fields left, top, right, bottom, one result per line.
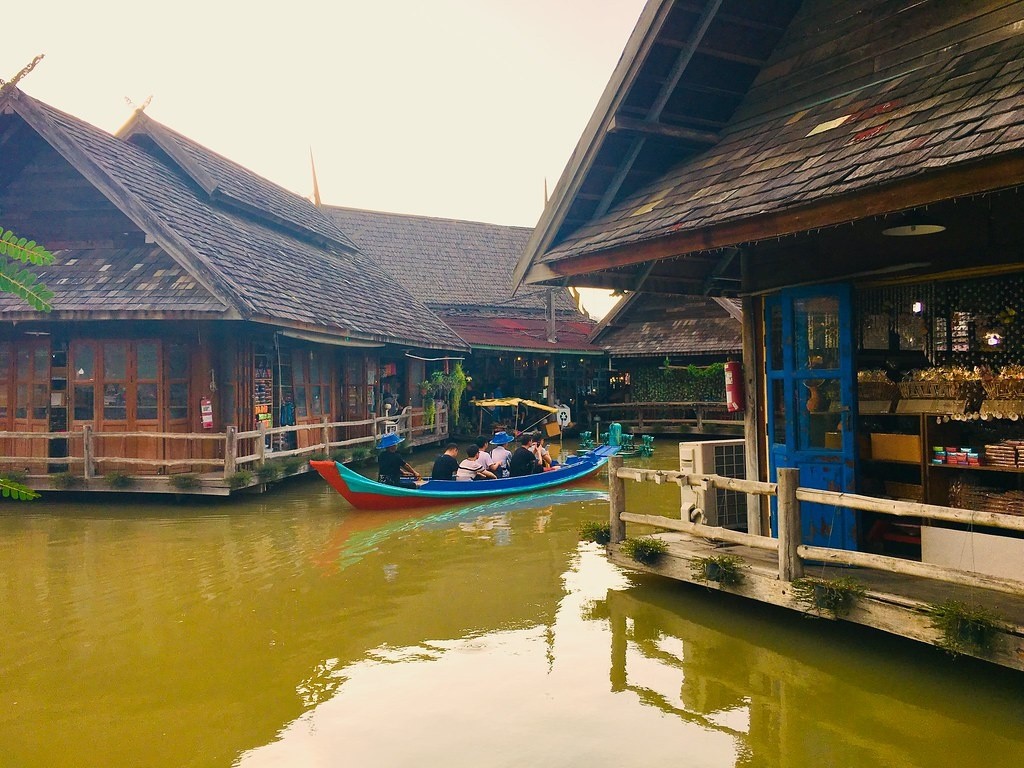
left=791, top=573, right=871, bottom=622
left=684, top=552, right=752, bottom=590
left=619, top=535, right=670, bottom=565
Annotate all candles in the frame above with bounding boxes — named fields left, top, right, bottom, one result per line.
left=915, top=597, right=1003, bottom=661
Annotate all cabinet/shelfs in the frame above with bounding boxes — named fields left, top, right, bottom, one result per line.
left=925, top=413, right=1024, bottom=528
left=809, top=410, right=924, bottom=543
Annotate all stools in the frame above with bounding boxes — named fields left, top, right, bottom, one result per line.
left=379, top=425, right=397, bottom=442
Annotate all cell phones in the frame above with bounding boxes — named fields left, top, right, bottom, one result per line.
left=546, top=445, right=550, bottom=451
left=537, top=441, right=541, bottom=448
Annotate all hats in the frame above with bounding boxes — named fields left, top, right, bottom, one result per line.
left=376, top=432, right=405, bottom=449
left=489, top=431, right=514, bottom=444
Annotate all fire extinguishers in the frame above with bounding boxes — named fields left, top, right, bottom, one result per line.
left=724, top=354, right=744, bottom=413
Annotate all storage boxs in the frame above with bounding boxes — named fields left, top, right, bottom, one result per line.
left=871, top=433, right=921, bottom=461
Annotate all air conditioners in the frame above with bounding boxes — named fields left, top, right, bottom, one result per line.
left=679, top=440, right=745, bottom=530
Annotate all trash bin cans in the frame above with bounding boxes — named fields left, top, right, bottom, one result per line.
left=554, top=403, right=570, bottom=427
left=379, top=421, right=398, bottom=435
left=200, top=395, right=213, bottom=428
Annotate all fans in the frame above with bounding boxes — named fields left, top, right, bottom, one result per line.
left=381, top=398, right=399, bottom=425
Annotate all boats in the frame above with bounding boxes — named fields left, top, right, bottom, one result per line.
left=309, top=395, right=624, bottom=512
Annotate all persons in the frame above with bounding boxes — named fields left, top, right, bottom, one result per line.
left=466, top=384, right=527, bottom=431
left=372, top=396, right=407, bottom=417
left=377, top=433, right=423, bottom=490
left=432, top=432, right=556, bottom=480
